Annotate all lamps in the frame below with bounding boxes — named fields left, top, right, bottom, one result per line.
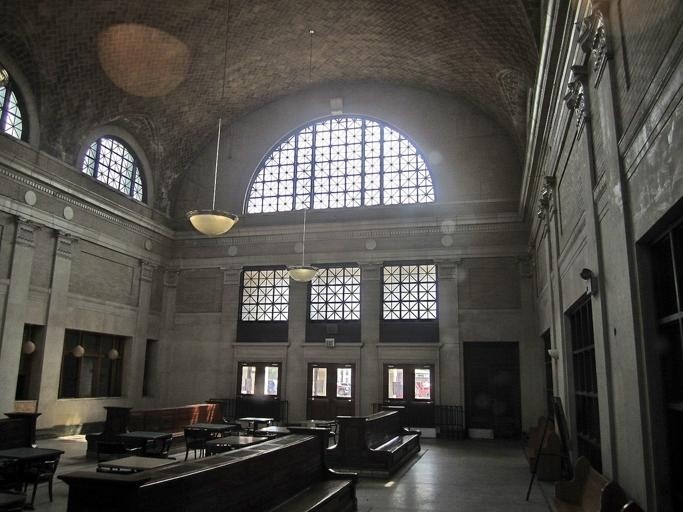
left=186, top=0, right=239, bottom=237
left=23, top=325, right=36, bottom=353
left=579, top=268, right=598, bottom=295
left=73, top=331, right=85, bottom=357
left=287, top=31, right=319, bottom=283
left=108, top=335, right=119, bottom=360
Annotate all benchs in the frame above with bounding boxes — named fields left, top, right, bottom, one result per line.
left=551, top=455, right=618, bottom=512
left=329, top=406, right=420, bottom=473
left=1, top=412, right=43, bottom=447
left=59, top=425, right=357, bottom=512
left=519, top=416, right=559, bottom=473
left=84, top=403, right=217, bottom=452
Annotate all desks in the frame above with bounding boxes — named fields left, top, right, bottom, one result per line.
left=536, top=448, right=562, bottom=480
left=97, top=455, right=170, bottom=474
left=119, top=432, right=173, bottom=458
left=184, top=416, right=291, bottom=455
left=1, top=447, right=65, bottom=512
left=302, top=419, right=338, bottom=447
